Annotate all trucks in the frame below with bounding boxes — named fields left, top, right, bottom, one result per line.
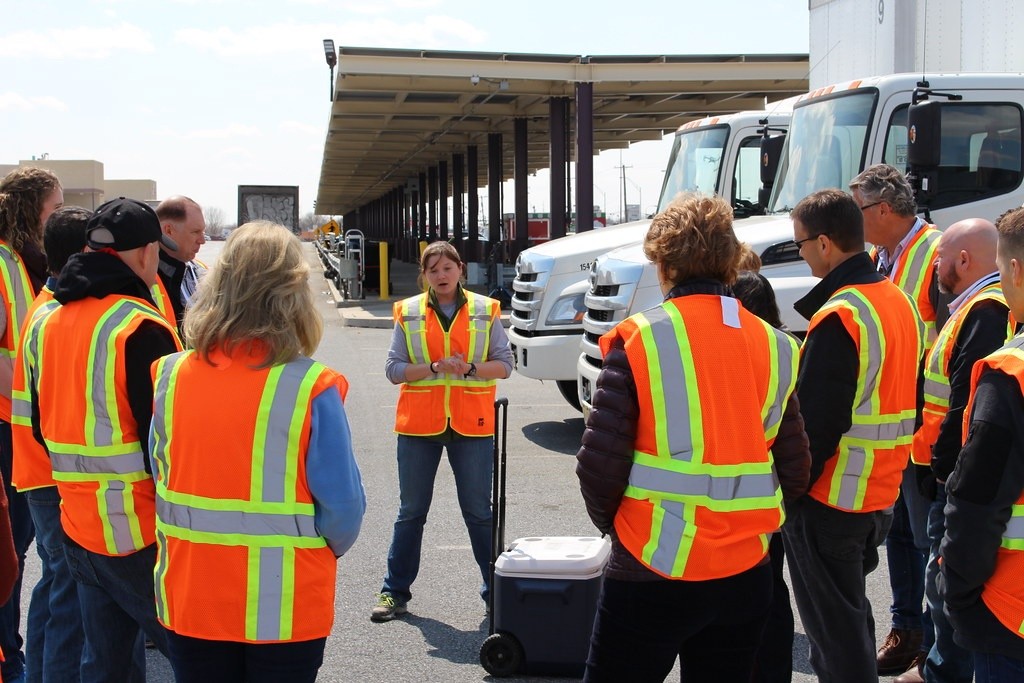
left=508, top=0, right=1024, bottom=432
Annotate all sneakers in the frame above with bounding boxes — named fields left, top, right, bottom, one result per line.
left=370, top=592, right=408, bottom=621
left=894, top=652, right=927, bottom=683
left=877, top=626, right=922, bottom=671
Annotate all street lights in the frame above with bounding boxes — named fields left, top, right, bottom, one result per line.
left=614, top=166, right=633, bottom=223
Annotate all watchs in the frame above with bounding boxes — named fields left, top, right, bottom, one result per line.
left=464, top=363, right=476, bottom=379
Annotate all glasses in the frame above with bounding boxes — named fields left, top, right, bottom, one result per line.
left=793, top=233, right=831, bottom=249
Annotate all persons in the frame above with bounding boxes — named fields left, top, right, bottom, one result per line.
left=848, top=163, right=951, bottom=673
left=0, top=167, right=65, bottom=683
left=148, top=219, right=367, bottom=683
left=913, top=207, right=1024, bottom=683
left=11, top=205, right=96, bottom=683
left=576, top=194, right=811, bottom=683
left=27, top=196, right=185, bottom=683
left=372, top=241, right=516, bottom=622
left=152, top=195, right=209, bottom=352
left=775, top=190, right=928, bottom=683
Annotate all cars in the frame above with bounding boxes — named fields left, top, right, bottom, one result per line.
left=205, top=230, right=229, bottom=241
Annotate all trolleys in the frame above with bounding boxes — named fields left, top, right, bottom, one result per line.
left=480, top=398, right=614, bottom=677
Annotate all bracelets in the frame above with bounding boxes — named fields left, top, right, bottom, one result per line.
left=430, top=362, right=438, bottom=373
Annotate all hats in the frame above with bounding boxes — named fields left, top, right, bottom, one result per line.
left=85, top=197, right=178, bottom=251
